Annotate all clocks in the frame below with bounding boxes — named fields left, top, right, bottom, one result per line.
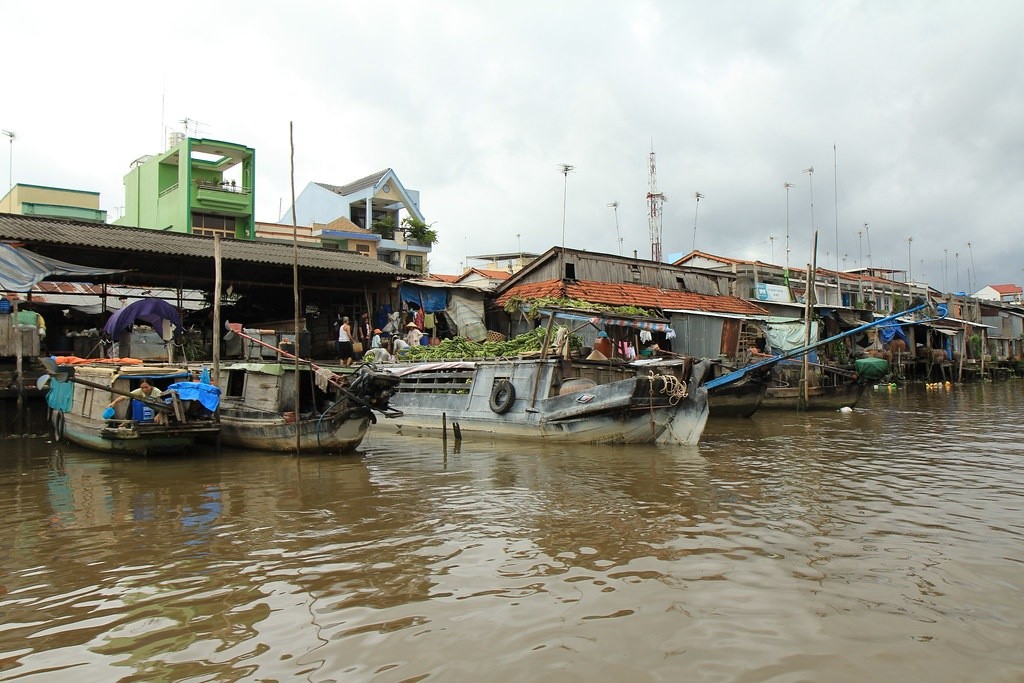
left=383, top=185, right=390, bottom=193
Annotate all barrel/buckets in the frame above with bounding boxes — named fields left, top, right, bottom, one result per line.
left=283, top=412, right=296, bottom=423
left=132, top=398, right=155, bottom=423
left=352, top=342, right=363, bottom=352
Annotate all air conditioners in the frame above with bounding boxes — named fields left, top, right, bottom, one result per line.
left=391, top=252, right=399, bottom=261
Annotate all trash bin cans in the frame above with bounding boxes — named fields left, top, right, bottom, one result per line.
left=420, top=333, right=429, bottom=346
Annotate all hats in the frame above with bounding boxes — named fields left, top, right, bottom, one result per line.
left=374, top=329, right=382, bottom=334
left=392, top=335, right=400, bottom=343
left=407, top=322, right=417, bottom=327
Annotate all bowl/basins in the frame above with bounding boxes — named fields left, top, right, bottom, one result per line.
left=640, top=350, right=652, bottom=357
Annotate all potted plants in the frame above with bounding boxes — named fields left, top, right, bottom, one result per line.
left=831, top=349, right=840, bottom=361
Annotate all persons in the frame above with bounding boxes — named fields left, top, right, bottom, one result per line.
left=357, top=312, right=370, bottom=358
left=106, top=379, right=162, bottom=427
left=339, top=317, right=357, bottom=366
left=407, top=322, right=423, bottom=346
left=393, top=337, right=409, bottom=354
left=372, top=329, right=382, bottom=348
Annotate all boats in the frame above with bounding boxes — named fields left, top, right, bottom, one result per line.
left=354, top=305, right=712, bottom=450
left=34, top=231, right=229, bottom=460
left=202, top=119, right=406, bottom=458
left=697, top=225, right=932, bottom=421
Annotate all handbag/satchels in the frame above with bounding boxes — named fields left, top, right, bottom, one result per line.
left=352, top=342, right=363, bottom=353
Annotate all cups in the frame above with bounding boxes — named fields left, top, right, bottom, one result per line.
left=102, top=407, right=115, bottom=420
